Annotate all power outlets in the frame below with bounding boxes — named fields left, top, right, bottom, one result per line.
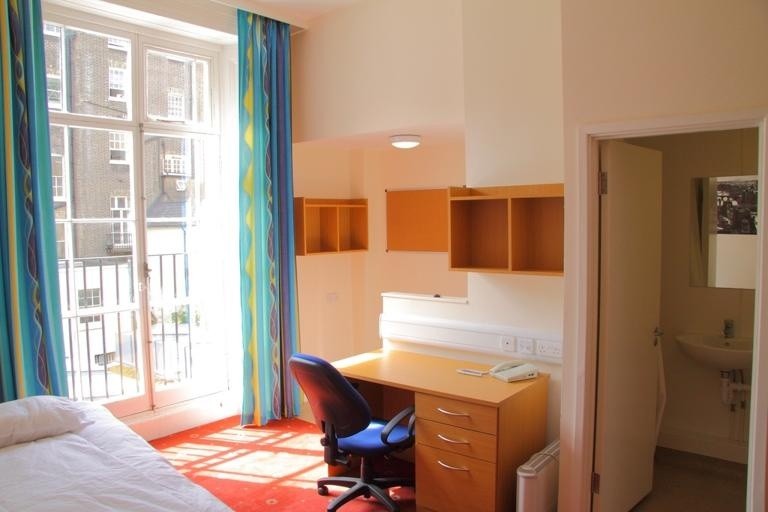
left=538, top=342, right=560, bottom=357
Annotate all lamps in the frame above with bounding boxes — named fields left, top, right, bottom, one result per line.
left=390, top=135, right=419, bottom=148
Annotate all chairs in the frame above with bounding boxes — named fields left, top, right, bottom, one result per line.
left=289, top=354, right=415, bottom=512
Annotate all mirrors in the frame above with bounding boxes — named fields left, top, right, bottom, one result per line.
left=689, top=174, right=759, bottom=289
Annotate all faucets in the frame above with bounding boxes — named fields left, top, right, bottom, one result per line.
left=719, top=319, right=733, bottom=338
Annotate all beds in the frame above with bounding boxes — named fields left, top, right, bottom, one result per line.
left=0, top=403, right=236, bottom=512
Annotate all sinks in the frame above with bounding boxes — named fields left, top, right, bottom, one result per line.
left=676, top=335, right=752, bottom=370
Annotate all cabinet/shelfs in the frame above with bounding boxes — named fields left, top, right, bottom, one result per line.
left=293, top=197, right=368, bottom=256
left=335, top=348, right=550, bottom=512
left=448, top=185, right=564, bottom=277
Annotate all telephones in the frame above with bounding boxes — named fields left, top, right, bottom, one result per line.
left=490, top=359, right=537, bottom=383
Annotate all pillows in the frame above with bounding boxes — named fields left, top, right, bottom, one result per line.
left=0, top=396, right=95, bottom=446
